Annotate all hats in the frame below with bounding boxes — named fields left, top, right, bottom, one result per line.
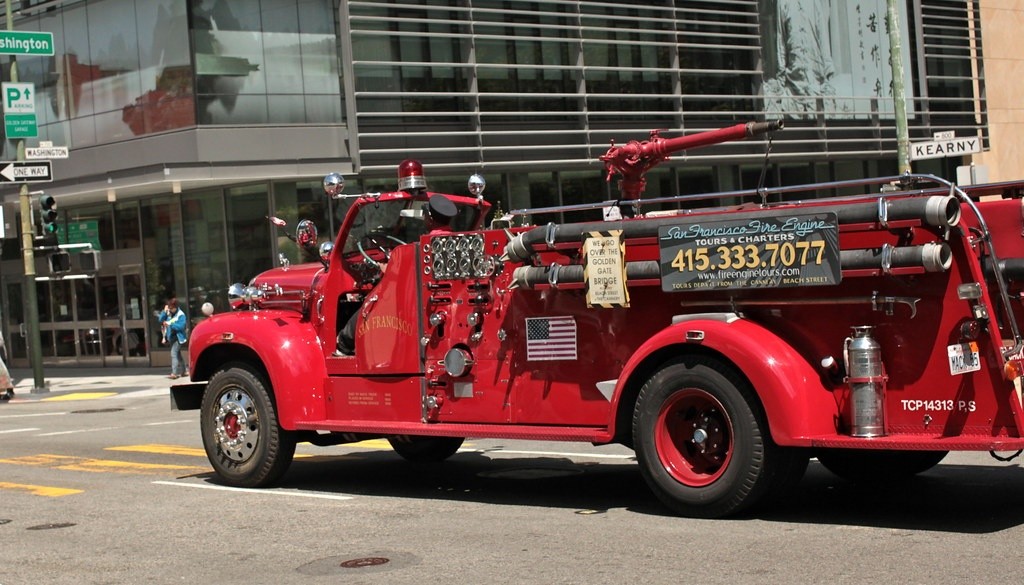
left=424, top=193, right=458, bottom=224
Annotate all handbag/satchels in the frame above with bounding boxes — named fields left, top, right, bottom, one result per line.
left=161, top=326, right=168, bottom=344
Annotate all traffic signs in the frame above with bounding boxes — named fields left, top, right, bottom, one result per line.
left=0, top=163, right=54, bottom=184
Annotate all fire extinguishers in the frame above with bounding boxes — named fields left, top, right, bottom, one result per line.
left=843, top=324, right=885, bottom=438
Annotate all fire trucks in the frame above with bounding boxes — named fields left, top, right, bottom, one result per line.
left=164, top=115, right=1024, bottom=518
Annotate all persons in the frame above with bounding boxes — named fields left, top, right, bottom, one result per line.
left=0, top=356, right=15, bottom=400
left=774, top=0, right=840, bottom=120
left=157, top=300, right=189, bottom=379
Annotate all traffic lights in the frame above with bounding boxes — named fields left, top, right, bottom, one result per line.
left=40, top=196, right=57, bottom=235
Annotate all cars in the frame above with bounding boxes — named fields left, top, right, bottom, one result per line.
left=61, top=302, right=134, bottom=343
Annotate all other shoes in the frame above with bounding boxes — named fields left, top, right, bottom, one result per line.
left=3, top=388, right=14, bottom=399
left=167, top=374, right=177, bottom=380
left=181, top=372, right=188, bottom=377
